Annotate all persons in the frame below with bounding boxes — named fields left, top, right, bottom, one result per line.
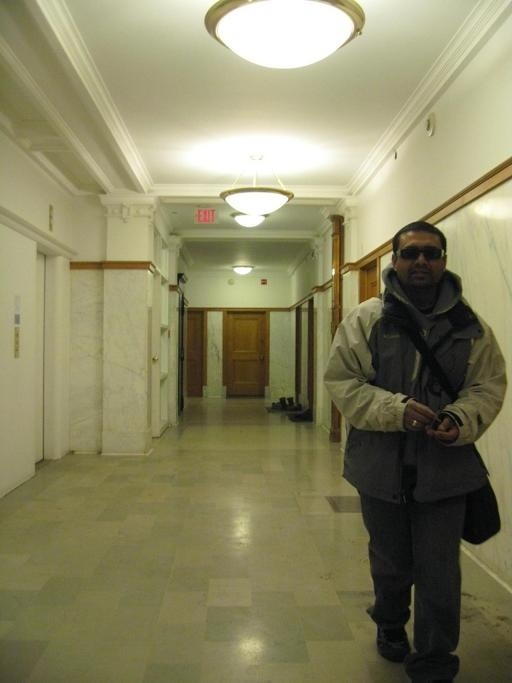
left=321, top=220, right=508, bottom=682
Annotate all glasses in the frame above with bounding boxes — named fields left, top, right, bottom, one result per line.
left=395, top=248, right=446, bottom=261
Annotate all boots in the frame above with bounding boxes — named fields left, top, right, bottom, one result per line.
left=272, top=397, right=312, bottom=422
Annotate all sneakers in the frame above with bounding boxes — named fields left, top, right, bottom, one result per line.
left=376, top=620, right=456, bottom=683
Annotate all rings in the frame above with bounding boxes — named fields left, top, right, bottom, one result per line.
left=411, top=419, right=417, bottom=427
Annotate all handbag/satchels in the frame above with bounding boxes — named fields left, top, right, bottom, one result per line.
left=458, top=444, right=501, bottom=544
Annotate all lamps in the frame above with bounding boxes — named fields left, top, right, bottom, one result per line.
left=230, top=212, right=269, bottom=228
left=204, top=0, right=366, bottom=71
left=219, top=187, right=294, bottom=217
left=232, top=266, right=254, bottom=275
